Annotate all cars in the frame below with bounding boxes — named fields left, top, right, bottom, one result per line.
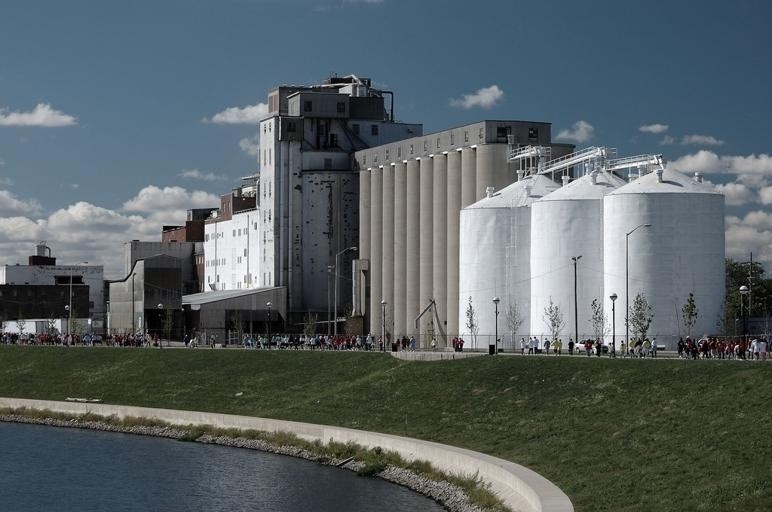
left=574, top=339, right=608, bottom=354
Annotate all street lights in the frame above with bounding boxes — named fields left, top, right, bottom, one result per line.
left=266, top=301, right=273, bottom=349
left=333, top=246, right=358, bottom=336
left=64, top=304, right=71, bottom=337
left=69, top=260, right=89, bottom=336
left=492, top=296, right=501, bottom=355
left=157, top=303, right=163, bottom=348
left=105, top=299, right=111, bottom=337
left=610, top=293, right=619, bottom=356
left=380, top=300, right=388, bottom=351
left=625, top=223, right=652, bottom=355
left=132, top=273, right=136, bottom=337
left=739, top=285, right=749, bottom=359
left=572, top=255, right=583, bottom=342
left=327, top=265, right=333, bottom=336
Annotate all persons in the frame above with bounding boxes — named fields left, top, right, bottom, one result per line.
left=0, top=332, right=159, bottom=347
left=585, top=337, right=614, bottom=358
left=620, top=338, right=657, bottom=358
left=395, top=336, right=416, bottom=352
left=432, top=337, right=437, bottom=351
left=521, top=336, right=574, bottom=355
left=183, top=334, right=215, bottom=348
left=453, top=336, right=464, bottom=352
left=678, top=335, right=769, bottom=361
left=243, top=333, right=383, bottom=352
